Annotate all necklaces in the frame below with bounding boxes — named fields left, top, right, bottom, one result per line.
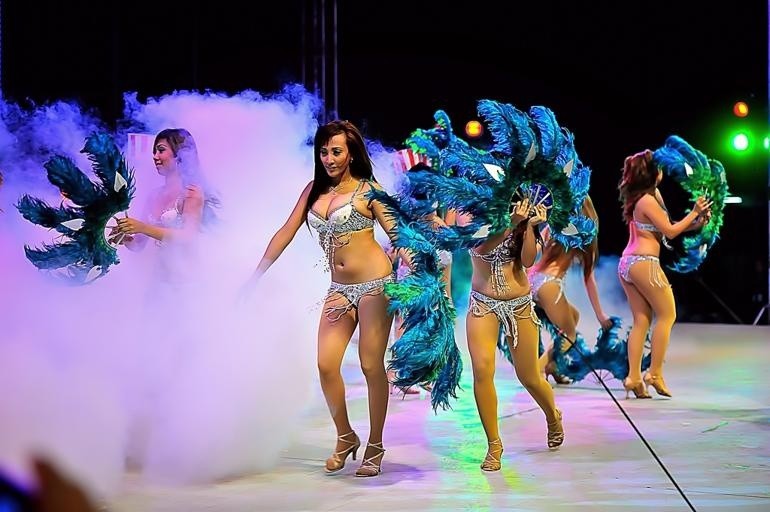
left=329, top=177, right=353, bottom=195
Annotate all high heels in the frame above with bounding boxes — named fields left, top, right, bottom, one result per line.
left=388, top=368, right=419, bottom=394
left=544, top=361, right=569, bottom=384
left=325, top=431, right=360, bottom=473
left=643, top=372, right=672, bottom=397
left=356, top=441, right=385, bottom=477
left=624, top=378, right=652, bottom=399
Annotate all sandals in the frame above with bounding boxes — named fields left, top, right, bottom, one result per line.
left=481, top=438, right=503, bottom=471
left=546, top=409, right=564, bottom=447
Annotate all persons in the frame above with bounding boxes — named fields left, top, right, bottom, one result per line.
left=453, top=197, right=567, bottom=472
left=246, top=117, right=419, bottom=478
left=388, top=159, right=458, bottom=398
left=105, top=126, right=221, bottom=475
left=526, top=191, right=612, bottom=386
left=614, top=147, right=715, bottom=401
